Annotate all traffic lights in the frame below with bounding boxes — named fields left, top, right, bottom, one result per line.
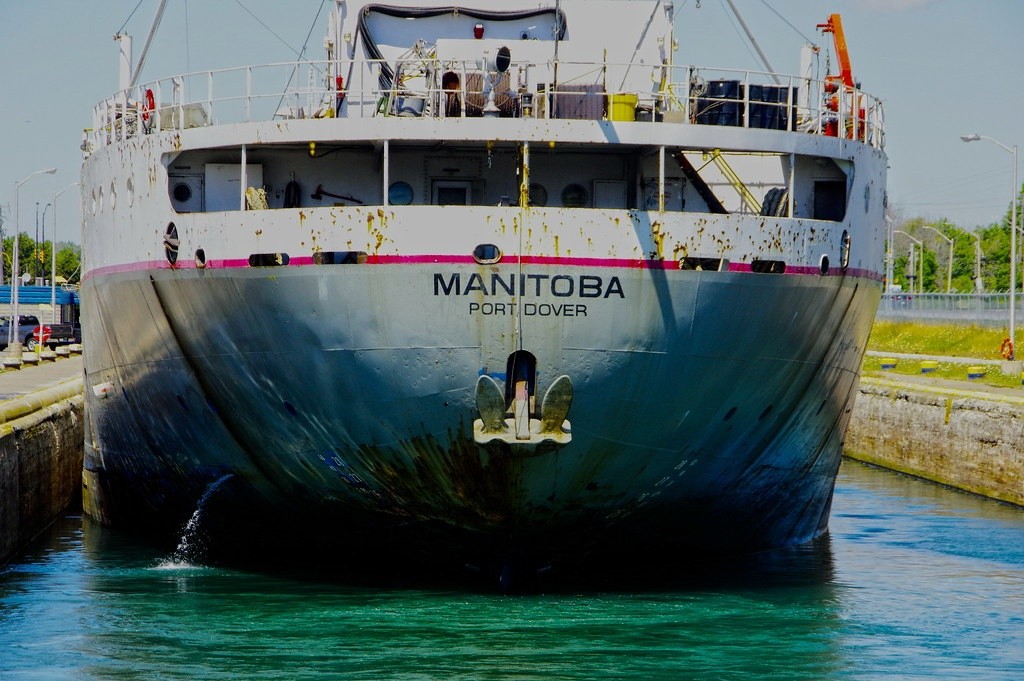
left=39, top=251, right=45, bottom=263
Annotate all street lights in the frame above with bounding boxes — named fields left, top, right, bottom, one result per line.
left=35, top=202, right=39, bottom=277
left=52, top=183, right=80, bottom=325
left=961, top=134, right=1018, bottom=361
left=893, top=230, right=923, bottom=293
left=14, top=168, right=57, bottom=342
left=945, top=222, right=981, bottom=294
left=42, top=203, right=51, bottom=283
left=922, top=226, right=954, bottom=293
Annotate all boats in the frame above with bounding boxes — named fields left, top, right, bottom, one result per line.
left=78, top=0, right=890, bottom=589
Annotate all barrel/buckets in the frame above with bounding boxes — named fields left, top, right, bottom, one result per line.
left=696, top=80, right=798, bottom=131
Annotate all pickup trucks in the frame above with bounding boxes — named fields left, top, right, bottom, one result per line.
left=0, top=314, right=74, bottom=352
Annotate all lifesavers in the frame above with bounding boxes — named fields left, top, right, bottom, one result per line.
left=1000, top=337, right=1013, bottom=359
left=142, top=89, right=154, bottom=120
left=33, top=325, right=50, bottom=343
